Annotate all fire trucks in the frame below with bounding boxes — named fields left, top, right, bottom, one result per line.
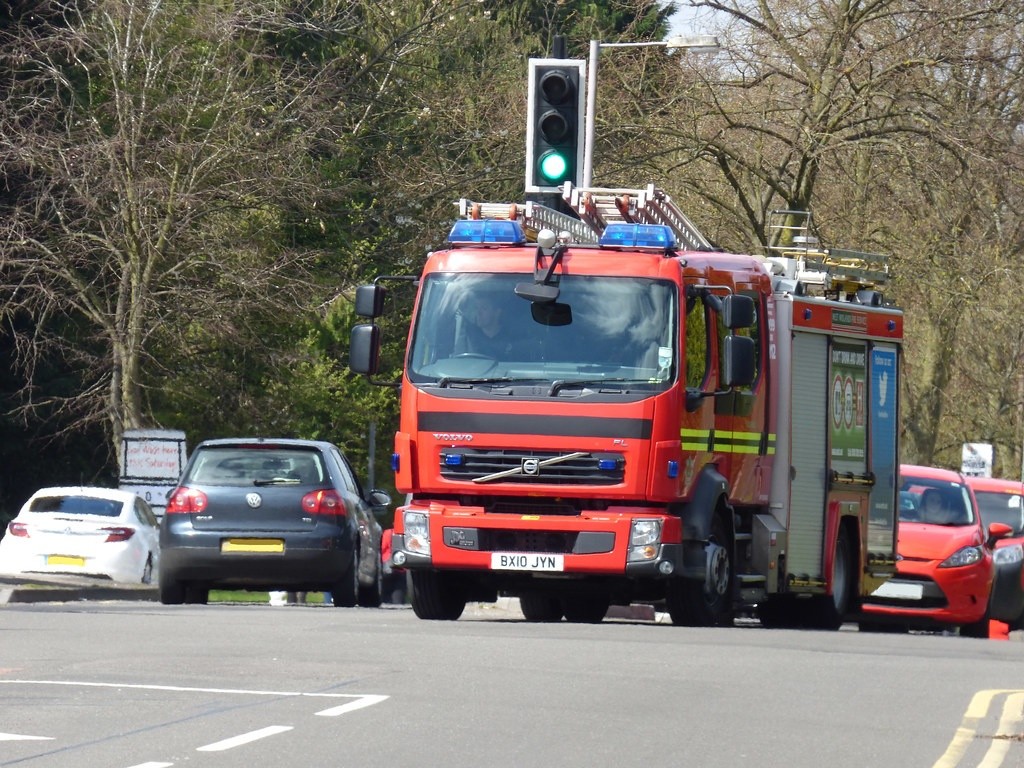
left=348, top=180, right=908, bottom=635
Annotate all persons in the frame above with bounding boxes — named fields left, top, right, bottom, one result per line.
left=915, top=488, right=955, bottom=522
left=461, top=288, right=528, bottom=364
left=288, top=589, right=331, bottom=604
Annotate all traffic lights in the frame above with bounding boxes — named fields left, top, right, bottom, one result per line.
left=524, top=57, right=584, bottom=196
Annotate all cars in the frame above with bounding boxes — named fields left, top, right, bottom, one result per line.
left=160, top=437, right=394, bottom=608
left=907, top=474, right=1024, bottom=629
left=846, top=464, right=1014, bottom=638
left=0, top=483, right=161, bottom=592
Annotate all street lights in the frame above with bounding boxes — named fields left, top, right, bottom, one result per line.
left=582, top=34, right=721, bottom=189
